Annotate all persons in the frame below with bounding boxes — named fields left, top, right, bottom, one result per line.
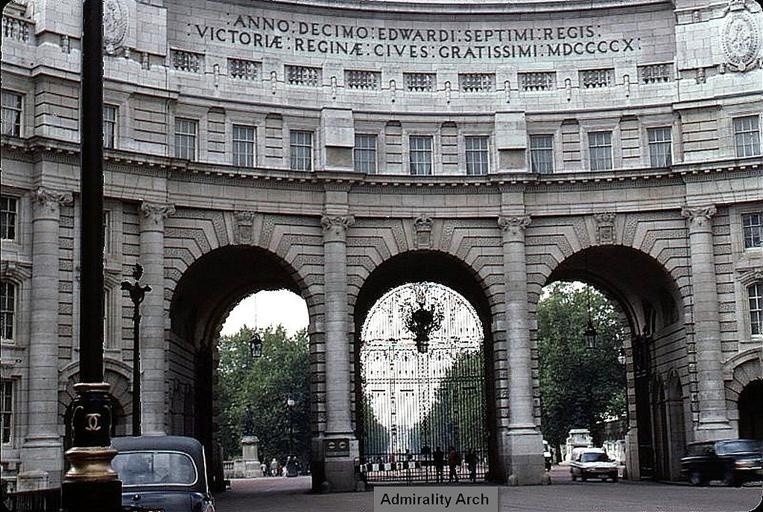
left=259, top=455, right=299, bottom=478
left=432, top=445, right=445, bottom=485
left=445, top=445, right=460, bottom=484
left=465, top=447, right=480, bottom=483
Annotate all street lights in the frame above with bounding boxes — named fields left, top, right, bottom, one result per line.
left=616, top=346, right=629, bottom=431
left=285, top=394, right=300, bottom=478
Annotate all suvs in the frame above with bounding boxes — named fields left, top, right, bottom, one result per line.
left=109, top=433, right=216, bottom=512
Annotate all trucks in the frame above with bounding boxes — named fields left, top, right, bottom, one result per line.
left=541, top=439, right=552, bottom=472
left=566, top=446, right=620, bottom=485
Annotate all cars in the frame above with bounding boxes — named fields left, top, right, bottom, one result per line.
left=679, top=437, right=763, bottom=489
